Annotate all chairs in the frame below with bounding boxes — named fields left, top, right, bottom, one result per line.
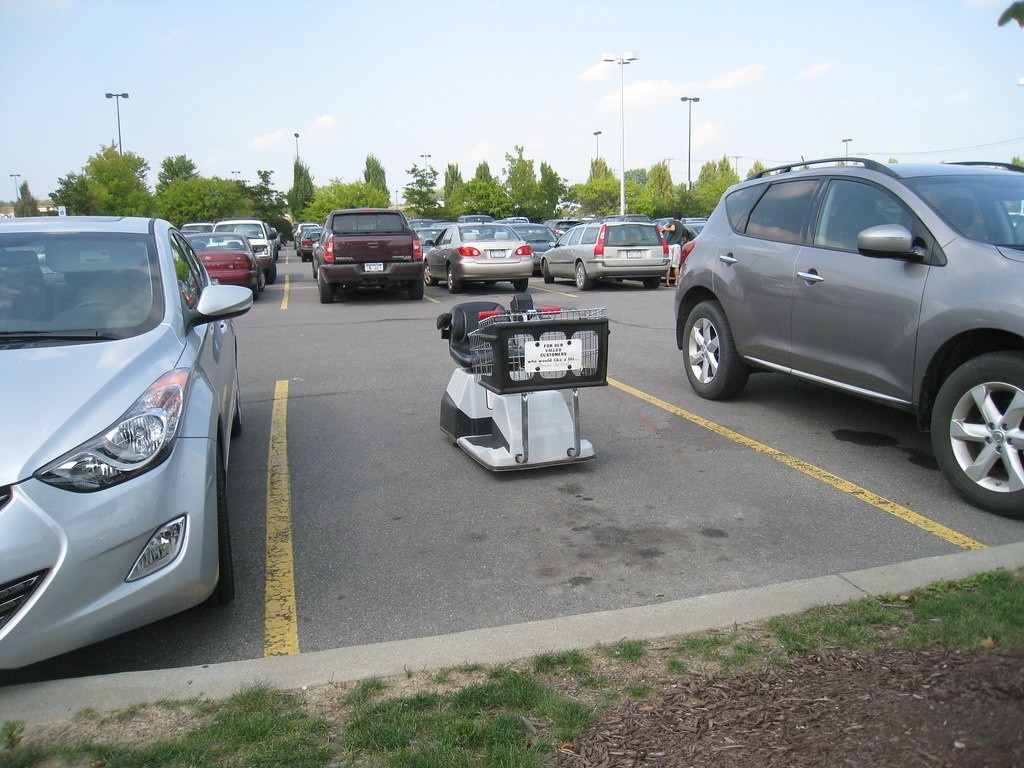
left=192, top=242, right=206, bottom=249
left=938, top=196, right=977, bottom=240
left=0, top=248, right=148, bottom=313
left=627, top=233, right=638, bottom=241
left=248, top=231, right=258, bottom=236
left=227, top=242, right=240, bottom=247
left=495, top=231, right=508, bottom=239
left=462, top=232, right=476, bottom=240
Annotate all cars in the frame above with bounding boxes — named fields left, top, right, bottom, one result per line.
left=423, top=220, right=534, bottom=293
left=539, top=219, right=670, bottom=290
left=179, top=223, right=215, bottom=239
left=0, top=214, right=254, bottom=671
left=183, top=231, right=267, bottom=301
left=264, top=214, right=708, bottom=267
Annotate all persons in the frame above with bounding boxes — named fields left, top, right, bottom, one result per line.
left=662, top=212, right=688, bottom=287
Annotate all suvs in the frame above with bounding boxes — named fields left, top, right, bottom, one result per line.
left=672, top=155, right=1024, bottom=522
left=310, top=208, right=425, bottom=304
left=205, top=219, right=279, bottom=286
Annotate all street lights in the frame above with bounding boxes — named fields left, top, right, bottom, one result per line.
left=105, top=92, right=129, bottom=156
left=842, top=139, right=852, bottom=166
left=231, top=171, right=241, bottom=181
left=594, top=130, right=602, bottom=160
left=10, top=174, right=21, bottom=202
left=420, top=154, right=431, bottom=180
left=681, top=96, right=701, bottom=191
left=293, top=133, right=299, bottom=160
left=602, top=51, right=640, bottom=215
